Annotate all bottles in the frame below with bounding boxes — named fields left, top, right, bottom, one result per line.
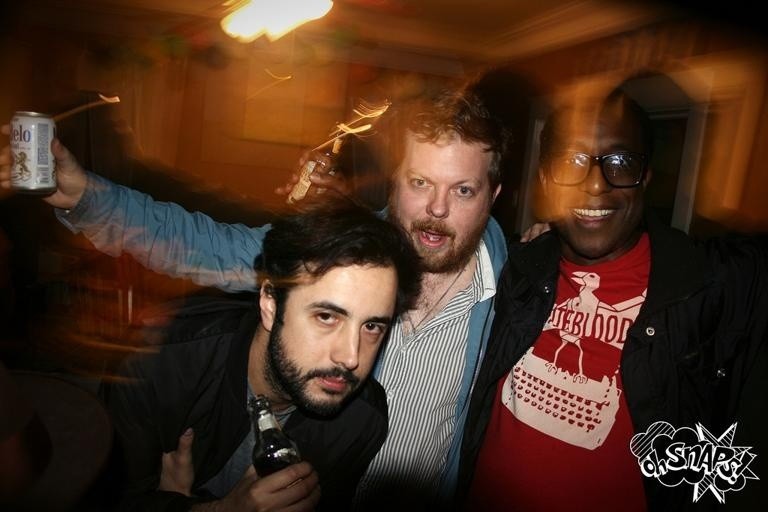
left=247, top=393, right=304, bottom=489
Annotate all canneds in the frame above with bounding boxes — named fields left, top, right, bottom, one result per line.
left=9, top=111, right=56, bottom=197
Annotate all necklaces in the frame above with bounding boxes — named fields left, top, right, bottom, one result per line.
left=401, top=258, right=471, bottom=338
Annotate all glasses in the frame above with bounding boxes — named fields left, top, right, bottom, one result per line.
left=542, top=150, right=653, bottom=188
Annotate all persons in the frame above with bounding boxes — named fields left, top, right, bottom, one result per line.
left=1, top=84, right=554, bottom=510
left=96, top=193, right=422, bottom=509
left=457, top=82, right=767, bottom=509
